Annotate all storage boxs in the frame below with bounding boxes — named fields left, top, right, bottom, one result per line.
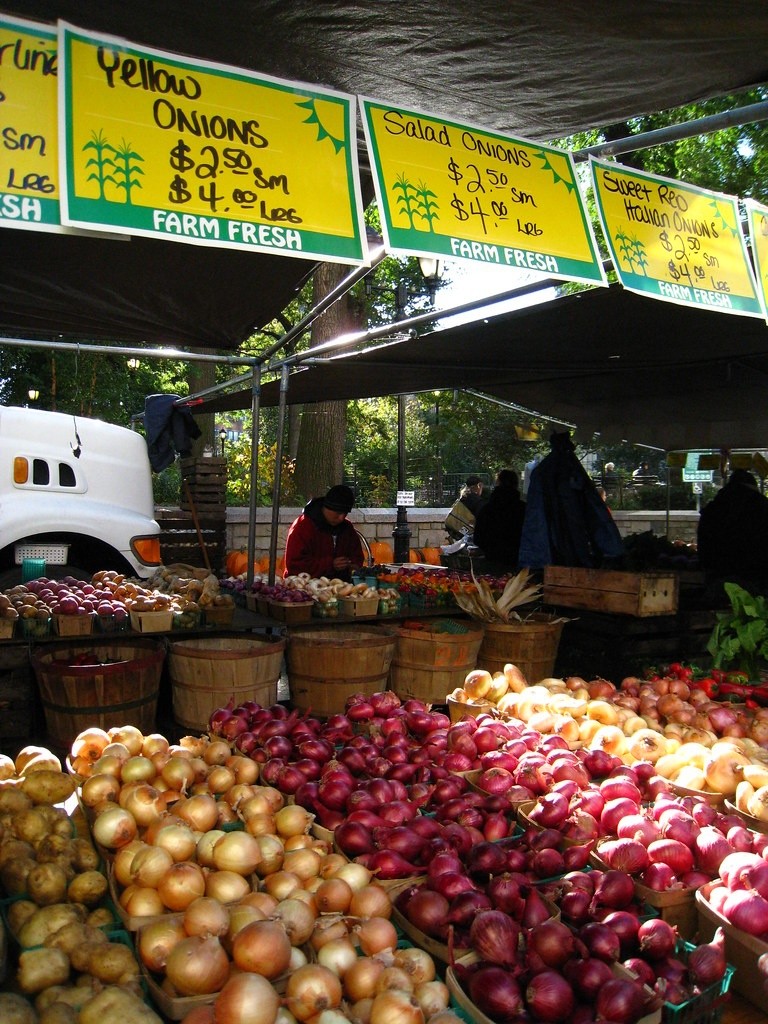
left=0, top=564, right=767, bottom=1023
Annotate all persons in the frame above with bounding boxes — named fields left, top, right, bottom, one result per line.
left=460, top=470, right=527, bottom=572
left=283, top=484, right=364, bottom=578
left=697, top=467, right=768, bottom=603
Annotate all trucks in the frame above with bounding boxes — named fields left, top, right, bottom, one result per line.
left=0, top=403, right=162, bottom=592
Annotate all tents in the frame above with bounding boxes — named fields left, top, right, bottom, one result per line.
left=142, top=260, right=768, bottom=586
left=0, top=0, right=768, bottom=588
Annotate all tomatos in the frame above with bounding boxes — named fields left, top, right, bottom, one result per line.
left=376, top=572, right=478, bottom=607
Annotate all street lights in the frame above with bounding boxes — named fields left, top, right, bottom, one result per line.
left=218, top=428, right=227, bottom=458
left=125, top=357, right=140, bottom=414
left=430, top=389, right=442, bottom=502
left=27, top=384, right=40, bottom=409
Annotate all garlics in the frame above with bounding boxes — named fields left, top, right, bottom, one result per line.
left=227, top=572, right=282, bottom=583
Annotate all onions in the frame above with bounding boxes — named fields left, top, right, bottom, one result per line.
left=284, top=573, right=400, bottom=616
left=207, top=693, right=768, bottom=1024
left=503, top=663, right=527, bottom=693
left=446, top=678, right=767, bottom=827
left=485, top=670, right=508, bottom=700
left=463, top=670, right=492, bottom=699
left=216, top=580, right=311, bottom=603
left=72, top=725, right=468, bottom=1024
left=398, top=567, right=513, bottom=590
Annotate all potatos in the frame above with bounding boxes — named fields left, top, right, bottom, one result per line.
left=0, top=576, right=126, bottom=620
left=0, top=745, right=162, bottom=1024
left=92, top=571, right=170, bottom=612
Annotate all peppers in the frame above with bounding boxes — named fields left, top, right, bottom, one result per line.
left=644, top=662, right=768, bottom=708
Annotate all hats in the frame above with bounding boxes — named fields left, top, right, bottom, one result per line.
left=322, top=484, right=355, bottom=513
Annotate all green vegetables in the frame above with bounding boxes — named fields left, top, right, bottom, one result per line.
left=594, top=530, right=698, bottom=573
left=706, top=582, right=768, bottom=668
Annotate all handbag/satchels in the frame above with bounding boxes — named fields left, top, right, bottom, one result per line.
left=444, top=498, right=477, bottom=535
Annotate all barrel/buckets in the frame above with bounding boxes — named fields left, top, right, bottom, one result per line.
left=30, top=640, right=164, bottom=751
left=476, top=610, right=563, bottom=687
left=167, top=632, right=287, bottom=728
left=388, top=616, right=486, bottom=706
left=282, top=623, right=399, bottom=716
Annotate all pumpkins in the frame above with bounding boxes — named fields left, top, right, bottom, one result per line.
left=225, top=543, right=450, bottom=580
